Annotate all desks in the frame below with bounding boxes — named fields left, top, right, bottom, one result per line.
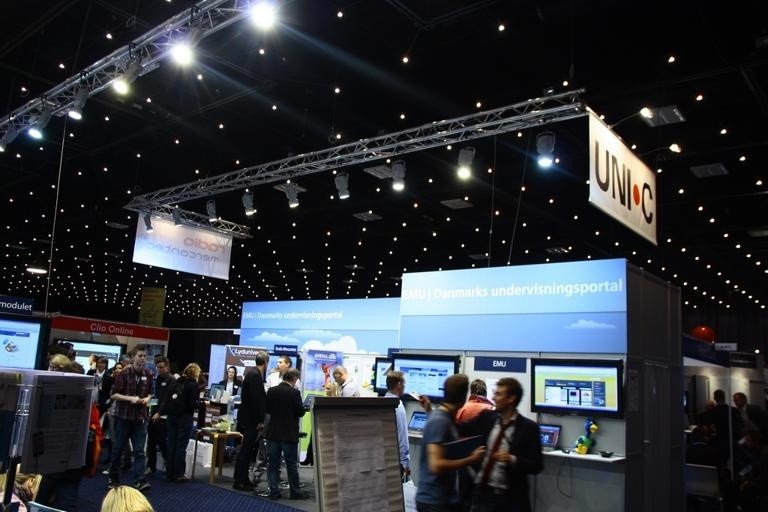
left=191, top=426, right=244, bottom=488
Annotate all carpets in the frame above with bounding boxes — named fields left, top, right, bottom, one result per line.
left=25, top=469, right=300, bottom=512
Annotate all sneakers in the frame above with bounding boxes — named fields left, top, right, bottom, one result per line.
left=101, top=464, right=191, bottom=482
left=133, top=479, right=151, bottom=493
left=106, top=476, right=122, bottom=488
left=270, top=493, right=282, bottom=501
left=288, top=492, right=311, bottom=501
left=231, top=473, right=263, bottom=491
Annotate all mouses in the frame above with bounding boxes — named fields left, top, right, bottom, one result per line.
left=563, top=448, right=570, bottom=454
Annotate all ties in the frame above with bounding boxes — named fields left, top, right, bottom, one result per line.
left=480, top=419, right=514, bottom=485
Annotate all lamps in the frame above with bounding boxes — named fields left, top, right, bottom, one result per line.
left=134, top=105, right=581, bottom=232
left=0, top=1, right=226, bottom=155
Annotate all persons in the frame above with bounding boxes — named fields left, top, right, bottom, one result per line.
left=18, top=343, right=209, bottom=512
left=217, top=352, right=359, bottom=500
left=416, top=374, right=545, bottom=512
left=699, top=390, right=767, bottom=511
left=381, top=371, right=411, bottom=481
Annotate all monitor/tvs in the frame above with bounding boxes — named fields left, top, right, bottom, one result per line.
left=530, top=357, right=624, bottom=419
left=53, top=337, right=127, bottom=373
left=0, top=312, right=52, bottom=371
left=391, top=352, right=460, bottom=403
left=375, top=357, right=392, bottom=391
left=209, top=383, right=226, bottom=398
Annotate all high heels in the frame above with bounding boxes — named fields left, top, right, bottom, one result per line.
left=299, top=459, right=314, bottom=467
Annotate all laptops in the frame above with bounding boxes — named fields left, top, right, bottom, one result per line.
left=538, top=424, right=561, bottom=452
left=408, top=411, right=428, bottom=432
left=304, top=393, right=323, bottom=409
